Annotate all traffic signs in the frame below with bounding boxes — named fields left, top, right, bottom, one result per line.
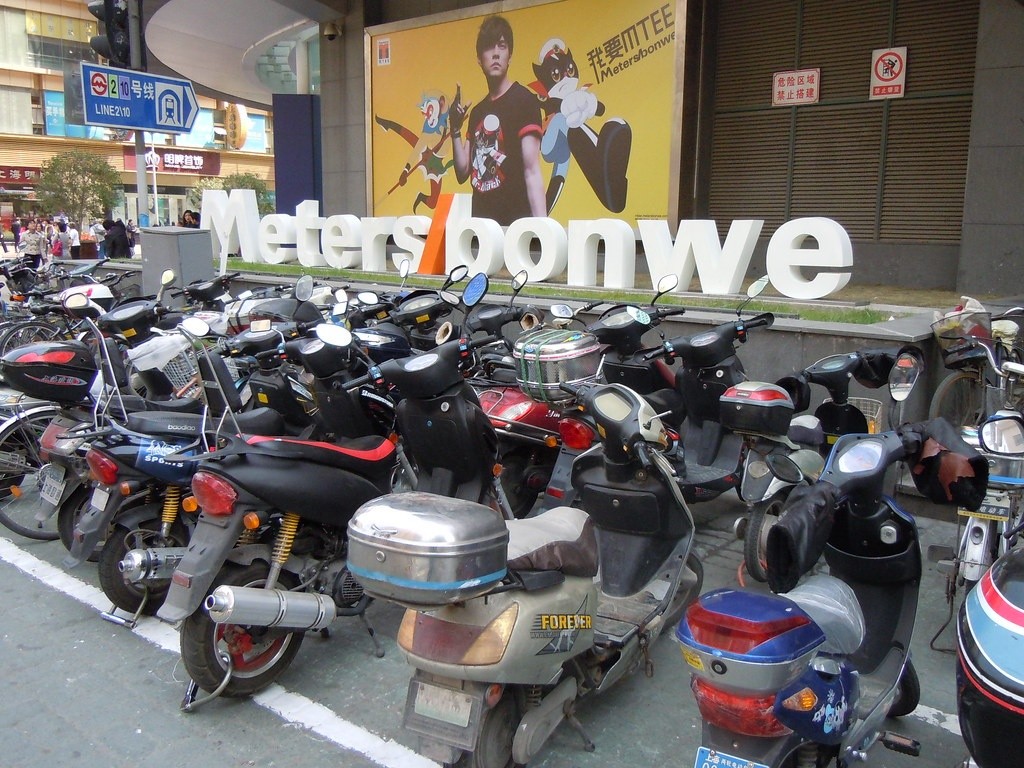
left=79, top=61, right=200, bottom=133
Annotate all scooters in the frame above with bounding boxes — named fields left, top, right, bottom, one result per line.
left=0, top=252, right=1023, bottom=768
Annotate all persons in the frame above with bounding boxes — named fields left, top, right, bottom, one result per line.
left=11, top=218, right=136, bottom=274
left=0, top=217, right=9, bottom=253
left=448, top=16, right=547, bottom=226
left=177, top=210, right=200, bottom=228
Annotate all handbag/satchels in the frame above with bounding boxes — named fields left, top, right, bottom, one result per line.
left=51, top=232, right=63, bottom=256
left=933, top=295, right=991, bottom=352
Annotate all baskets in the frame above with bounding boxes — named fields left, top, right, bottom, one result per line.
left=822, top=395, right=882, bottom=435
left=161, top=344, right=240, bottom=398
left=930, top=311, right=993, bottom=369
left=110, top=283, right=141, bottom=309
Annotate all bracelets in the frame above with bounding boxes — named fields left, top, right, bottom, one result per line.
left=453, top=133, right=461, bottom=138
